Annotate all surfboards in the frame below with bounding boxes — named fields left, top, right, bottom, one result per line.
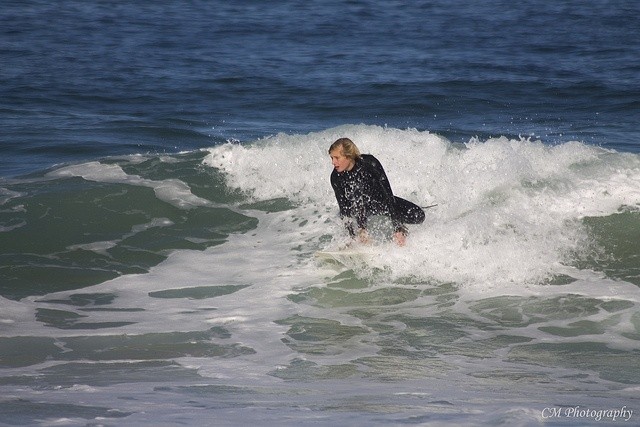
left=315, top=247, right=394, bottom=256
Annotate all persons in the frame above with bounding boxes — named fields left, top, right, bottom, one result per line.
left=328, top=138, right=426, bottom=247
